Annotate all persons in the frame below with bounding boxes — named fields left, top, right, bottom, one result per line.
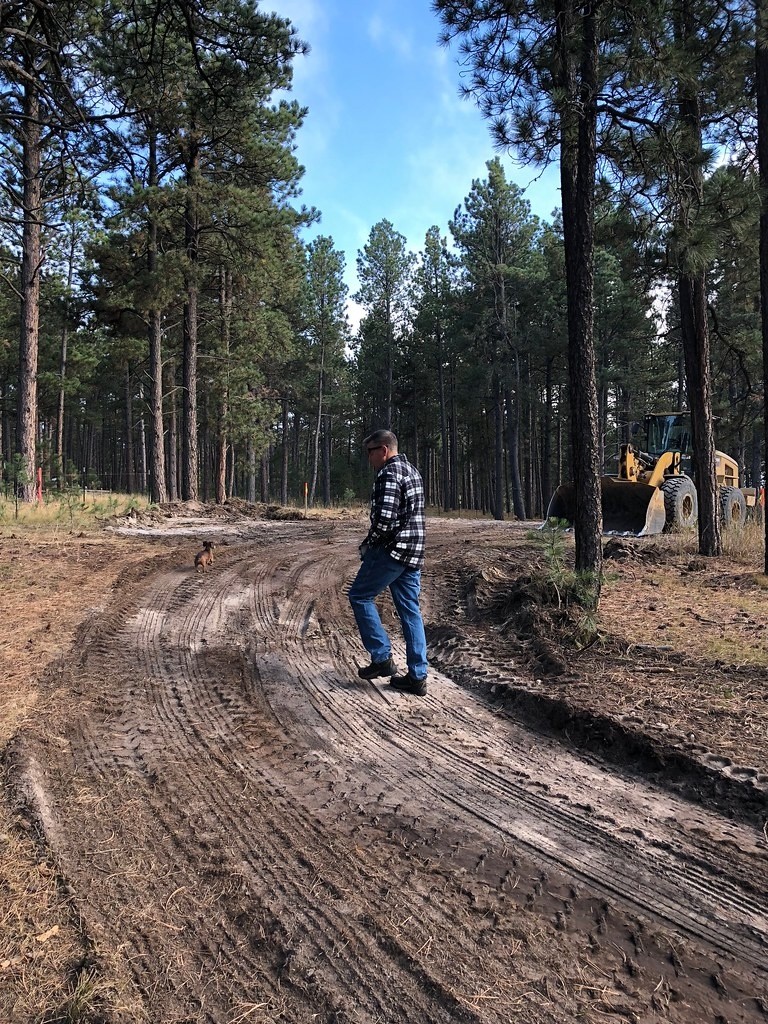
left=349, top=429, right=428, bottom=697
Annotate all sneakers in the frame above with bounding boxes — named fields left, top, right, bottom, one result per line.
left=358, top=653, right=397, bottom=680
left=389, top=673, right=427, bottom=696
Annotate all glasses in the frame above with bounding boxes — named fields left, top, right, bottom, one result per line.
left=367, top=445, right=388, bottom=454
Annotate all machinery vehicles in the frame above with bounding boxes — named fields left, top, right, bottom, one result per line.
left=538, top=409, right=756, bottom=542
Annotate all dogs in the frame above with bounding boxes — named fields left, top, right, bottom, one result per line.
left=194, top=541, right=216, bottom=573
